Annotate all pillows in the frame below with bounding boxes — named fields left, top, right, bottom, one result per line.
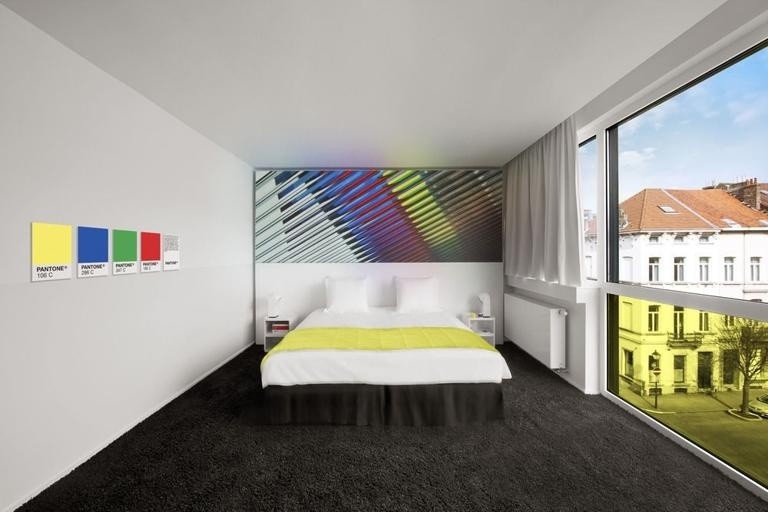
left=324, top=278, right=369, bottom=315
left=394, top=277, right=439, bottom=313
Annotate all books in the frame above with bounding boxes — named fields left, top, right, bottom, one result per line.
left=271, top=321, right=288, bottom=335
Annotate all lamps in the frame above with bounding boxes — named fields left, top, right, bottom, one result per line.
left=265, top=291, right=282, bottom=317
left=477, top=292, right=490, bottom=318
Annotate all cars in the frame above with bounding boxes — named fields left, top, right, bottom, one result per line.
left=749, top=393, right=768, bottom=418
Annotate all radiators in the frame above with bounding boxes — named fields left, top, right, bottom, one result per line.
left=502, top=292, right=567, bottom=370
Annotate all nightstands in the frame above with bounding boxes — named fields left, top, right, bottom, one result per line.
left=264, top=312, right=298, bottom=352
left=461, top=314, right=495, bottom=348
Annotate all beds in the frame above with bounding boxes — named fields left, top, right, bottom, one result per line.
left=260, top=306, right=513, bottom=425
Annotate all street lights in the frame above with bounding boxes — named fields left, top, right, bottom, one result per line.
left=652, top=352, right=661, bottom=407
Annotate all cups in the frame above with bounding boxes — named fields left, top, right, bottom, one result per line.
left=478, top=313, right=483, bottom=317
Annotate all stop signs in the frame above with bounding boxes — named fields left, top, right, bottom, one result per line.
left=654, top=368, right=661, bottom=376
